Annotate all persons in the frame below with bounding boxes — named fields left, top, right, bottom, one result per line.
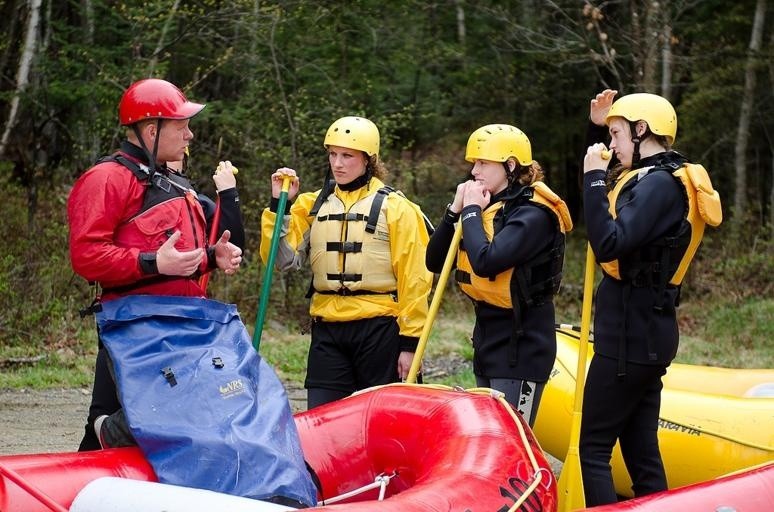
left=259, top=115, right=436, bottom=411
left=575, top=88, right=724, bottom=507
left=426, top=124, right=574, bottom=429
left=163, top=145, right=248, bottom=259
left=63, top=76, right=246, bottom=452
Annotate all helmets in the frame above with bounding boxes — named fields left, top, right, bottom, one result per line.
left=185, top=146, right=189, bottom=157
left=604, top=92, right=678, bottom=146
left=464, top=124, right=533, bottom=166
left=119, top=78, right=206, bottom=126
left=323, top=116, right=380, bottom=166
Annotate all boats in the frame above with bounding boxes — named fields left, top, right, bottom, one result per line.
left=571, top=463, right=773, bottom=512
left=490, top=323, right=773, bottom=497
left=0, top=382, right=557, bottom=512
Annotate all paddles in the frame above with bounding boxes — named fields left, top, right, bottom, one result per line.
left=556, top=150, right=611, bottom=512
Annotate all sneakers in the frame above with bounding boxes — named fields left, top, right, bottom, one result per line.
left=93, top=414, right=110, bottom=448
